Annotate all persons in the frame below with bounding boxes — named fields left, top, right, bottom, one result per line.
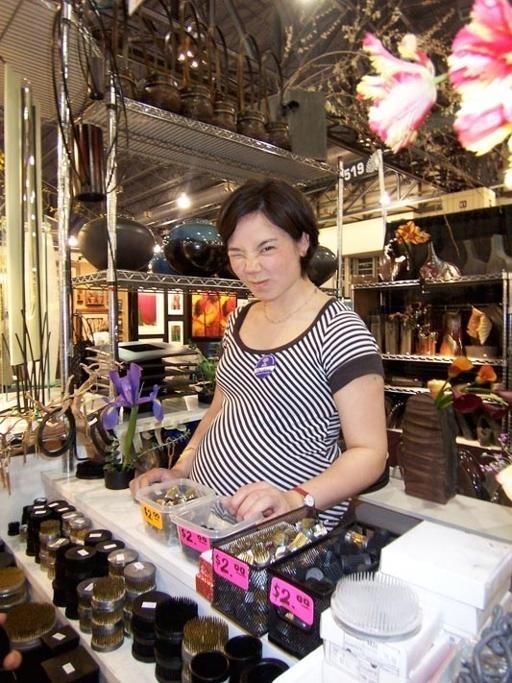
left=129, top=178, right=387, bottom=536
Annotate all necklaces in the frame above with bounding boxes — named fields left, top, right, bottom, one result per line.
left=263, top=287, right=317, bottom=324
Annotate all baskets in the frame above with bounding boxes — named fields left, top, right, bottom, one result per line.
left=211, top=504, right=345, bottom=638
left=264, top=520, right=401, bottom=658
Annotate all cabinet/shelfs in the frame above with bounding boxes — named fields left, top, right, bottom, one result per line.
left=349, top=268, right=512, bottom=453
left=60, top=88, right=344, bottom=474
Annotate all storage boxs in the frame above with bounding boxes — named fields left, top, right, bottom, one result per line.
left=134, top=477, right=216, bottom=548
left=379, top=521, right=512, bottom=637
left=169, top=495, right=265, bottom=567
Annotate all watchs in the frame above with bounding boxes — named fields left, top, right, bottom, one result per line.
left=291, top=488, right=315, bottom=507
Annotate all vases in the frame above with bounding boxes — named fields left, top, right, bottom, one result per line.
left=78, top=213, right=156, bottom=271
left=308, top=244, right=337, bottom=287
left=400, top=397, right=458, bottom=503
left=164, top=218, right=228, bottom=276
left=104, top=466, right=130, bottom=490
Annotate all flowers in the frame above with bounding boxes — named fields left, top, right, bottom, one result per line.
left=426, top=354, right=512, bottom=419
left=100, top=363, right=163, bottom=471
